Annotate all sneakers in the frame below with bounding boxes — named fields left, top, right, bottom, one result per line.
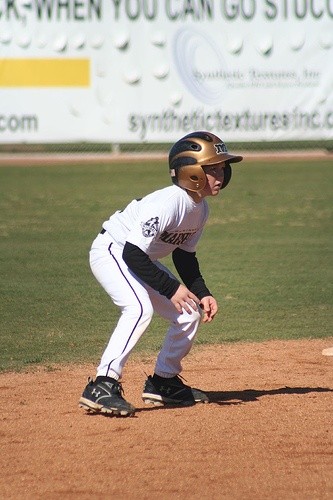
left=141, top=373, right=209, bottom=405
left=78, top=376, right=135, bottom=418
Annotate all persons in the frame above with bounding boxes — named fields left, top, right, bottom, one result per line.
left=79, top=131, right=244, bottom=418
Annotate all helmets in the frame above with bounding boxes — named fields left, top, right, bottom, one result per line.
left=169, top=132, right=243, bottom=192
